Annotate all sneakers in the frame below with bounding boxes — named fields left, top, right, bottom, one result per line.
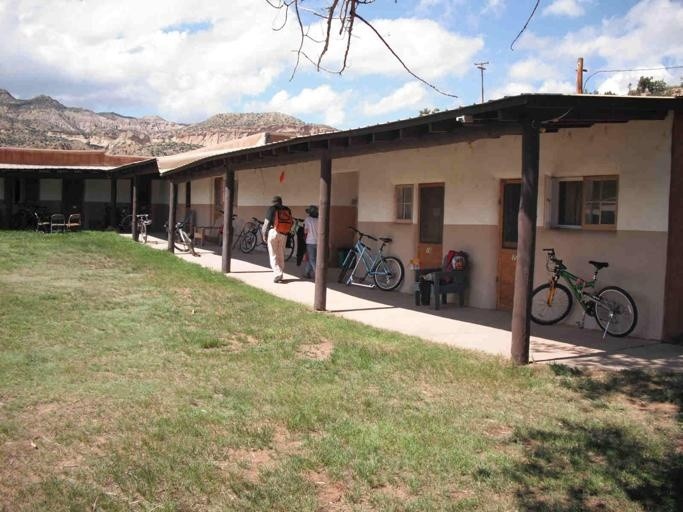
left=273, top=275, right=282, bottom=283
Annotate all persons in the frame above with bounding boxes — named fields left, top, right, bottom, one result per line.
left=299, top=204, right=318, bottom=281
left=260, top=195, right=291, bottom=284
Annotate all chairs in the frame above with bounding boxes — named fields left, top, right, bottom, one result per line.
left=413, top=250, right=468, bottom=310
left=33, top=212, right=82, bottom=234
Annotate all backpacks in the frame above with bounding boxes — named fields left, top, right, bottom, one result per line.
left=271, top=205, right=294, bottom=235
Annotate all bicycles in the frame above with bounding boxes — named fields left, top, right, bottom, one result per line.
left=532, top=246, right=640, bottom=342
left=8, top=198, right=238, bottom=257
left=337, top=226, right=405, bottom=292
left=284, top=218, right=310, bottom=263
left=241, top=216, right=271, bottom=255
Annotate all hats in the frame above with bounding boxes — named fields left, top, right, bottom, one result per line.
left=303, top=205, right=319, bottom=217
